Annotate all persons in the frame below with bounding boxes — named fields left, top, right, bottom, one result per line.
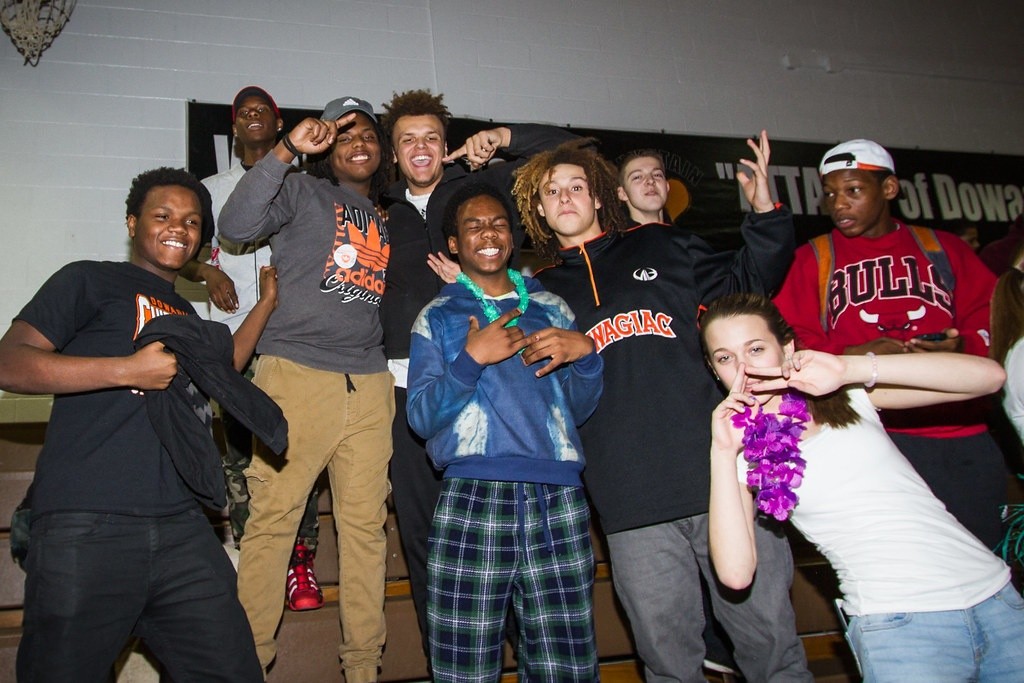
left=773, top=141, right=1009, bottom=567
left=426, top=135, right=815, bottom=682
left=616, top=151, right=734, bottom=673
left=377, top=86, right=596, bottom=683
left=0, top=167, right=276, bottom=683
left=406, top=184, right=604, bottom=683
left=200, top=86, right=322, bottom=612
left=698, top=292, right=1024, bottom=683
left=217, top=98, right=397, bottom=683
left=990, top=217, right=1024, bottom=442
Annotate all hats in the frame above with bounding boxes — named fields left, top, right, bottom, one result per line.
left=232, top=86, right=280, bottom=123
left=817, top=139, right=907, bottom=199
left=320, top=96, right=377, bottom=130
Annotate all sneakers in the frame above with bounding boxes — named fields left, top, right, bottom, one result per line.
left=285, top=546, right=325, bottom=610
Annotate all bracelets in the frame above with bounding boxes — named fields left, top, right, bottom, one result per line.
left=283, top=133, right=301, bottom=156
left=863, top=351, right=876, bottom=387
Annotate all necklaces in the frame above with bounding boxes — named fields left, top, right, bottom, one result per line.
left=730, top=390, right=811, bottom=521
left=457, top=267, right=528, bottom=354
